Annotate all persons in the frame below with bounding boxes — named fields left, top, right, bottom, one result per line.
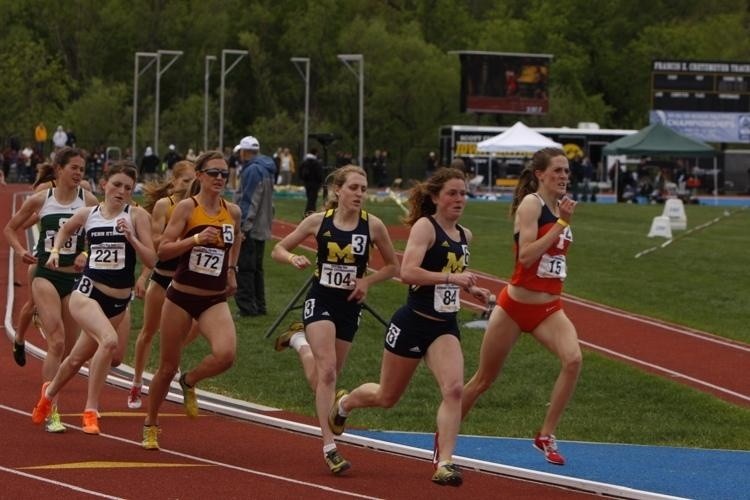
left=327, top=168, right=493, bottom=485
left=269, top=164, right=401, bottom=474
left=434, top=145, right=583, bottom=469
left=2, top=116, right=273, bottom=450
left=271, top=149, right=692, bottom=207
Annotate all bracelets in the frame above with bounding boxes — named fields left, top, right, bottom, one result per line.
left=445, top=272, right=450, bottom=285
left=289, top=254, right=296, bottom=263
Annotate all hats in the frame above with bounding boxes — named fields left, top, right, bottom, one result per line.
left=232, top=135, right=261, bottom=154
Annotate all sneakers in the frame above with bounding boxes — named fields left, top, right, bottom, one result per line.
left=532, top=432, right=565, bottom=464
left=44, top=411, right=66, bottom=433
left=82, top=409, right=100, bottom=434
left=142, top=424, right=161, bottom=450
left=127, top=381, right=142, bottom=409
left=274, top=320, right=305, bottom=352
left=12, top=338, right=26, bottom=366
left=431, top=464, right=464, bottom=485
left=179, top=372, right=199, bottom=418
left=31, top=381, right=53, bottom=426
left=328, top=388, right=350, bottom=436
left=432, top=431, right=440, bottom=473
left=324, top=447, right=352, bottom=474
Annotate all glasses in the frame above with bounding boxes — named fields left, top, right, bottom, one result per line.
left=199, top=167, right=230, bottom=179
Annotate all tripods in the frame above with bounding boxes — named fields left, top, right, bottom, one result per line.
left=264, top=205, right=392, bottom=339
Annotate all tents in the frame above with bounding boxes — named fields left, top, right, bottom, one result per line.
left=478, top=122, right=563, bottom=192
left=603, top=121, right=719, bottom=208
